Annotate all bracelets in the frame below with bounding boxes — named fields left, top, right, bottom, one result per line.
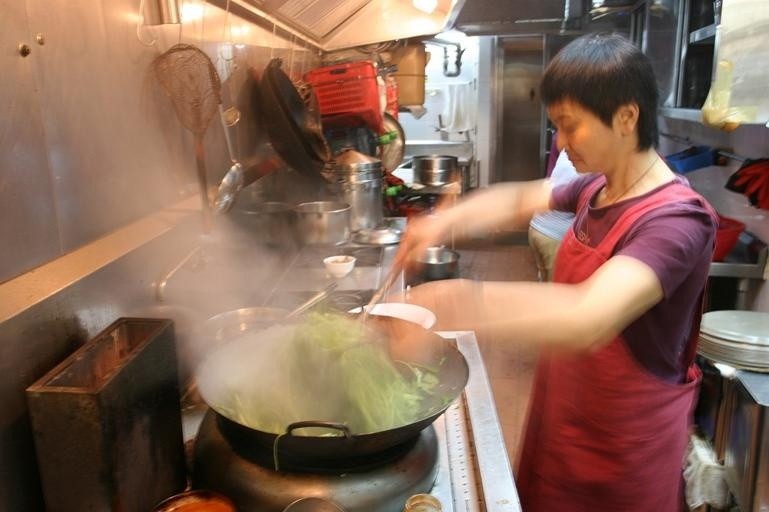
left=405, top=282, right=411, bottom=307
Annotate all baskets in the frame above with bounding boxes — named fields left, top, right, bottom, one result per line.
left=711, top=216, right=745, bottom=262
left=303, top=60, right=384, bottom=139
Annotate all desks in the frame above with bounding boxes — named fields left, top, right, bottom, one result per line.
left=700, top=229, right=769, bottom=310
left=696, top=359, right=768, bottom=511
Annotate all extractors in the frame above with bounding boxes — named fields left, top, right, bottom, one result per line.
left=205, top=0, right=466, bottom=58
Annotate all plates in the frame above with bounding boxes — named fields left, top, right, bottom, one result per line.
left=348, top=302, right=437, bottom=331
left=696, top=310, right=769, bottom=373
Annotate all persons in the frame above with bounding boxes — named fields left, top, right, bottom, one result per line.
left=528, top=145, right=731, bottom=283
left=385, top=30, right=719, bottom=510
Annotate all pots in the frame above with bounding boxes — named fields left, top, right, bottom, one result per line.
left=257, top=58, right=337, bottom=185
left=320, top=149, right=384, bottom=233
left=194, top=294, right=469, bottom=457
left=293, top=201, right=352, bottom=247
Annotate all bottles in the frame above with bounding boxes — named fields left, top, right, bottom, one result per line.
left=403, top=493, right=443, bottom=512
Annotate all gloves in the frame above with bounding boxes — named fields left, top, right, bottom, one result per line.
left=735, top=161, right=769, bottom=211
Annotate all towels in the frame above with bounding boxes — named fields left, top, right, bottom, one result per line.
left=442, top=82, right=474, bottom=133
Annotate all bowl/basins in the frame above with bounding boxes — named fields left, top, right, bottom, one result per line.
left=414, top=249, right=460, bottom=280
left=323, top=255, right=357, bottom=278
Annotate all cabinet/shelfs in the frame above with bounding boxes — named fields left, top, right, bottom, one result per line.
left=629, top=0, right=768, bottom=127
